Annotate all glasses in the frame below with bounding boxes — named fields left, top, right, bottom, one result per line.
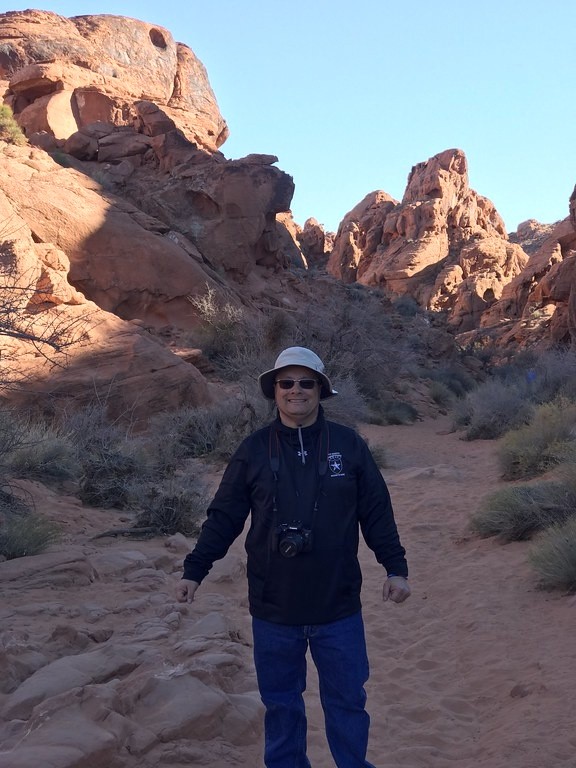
left=275, top=379, right=315, bottom=390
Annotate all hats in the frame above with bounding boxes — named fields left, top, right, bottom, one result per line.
left=257, top=346, right=339, bottom=401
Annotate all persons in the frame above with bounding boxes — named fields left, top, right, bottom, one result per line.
left=176, top=347, right=411, bottom=768
